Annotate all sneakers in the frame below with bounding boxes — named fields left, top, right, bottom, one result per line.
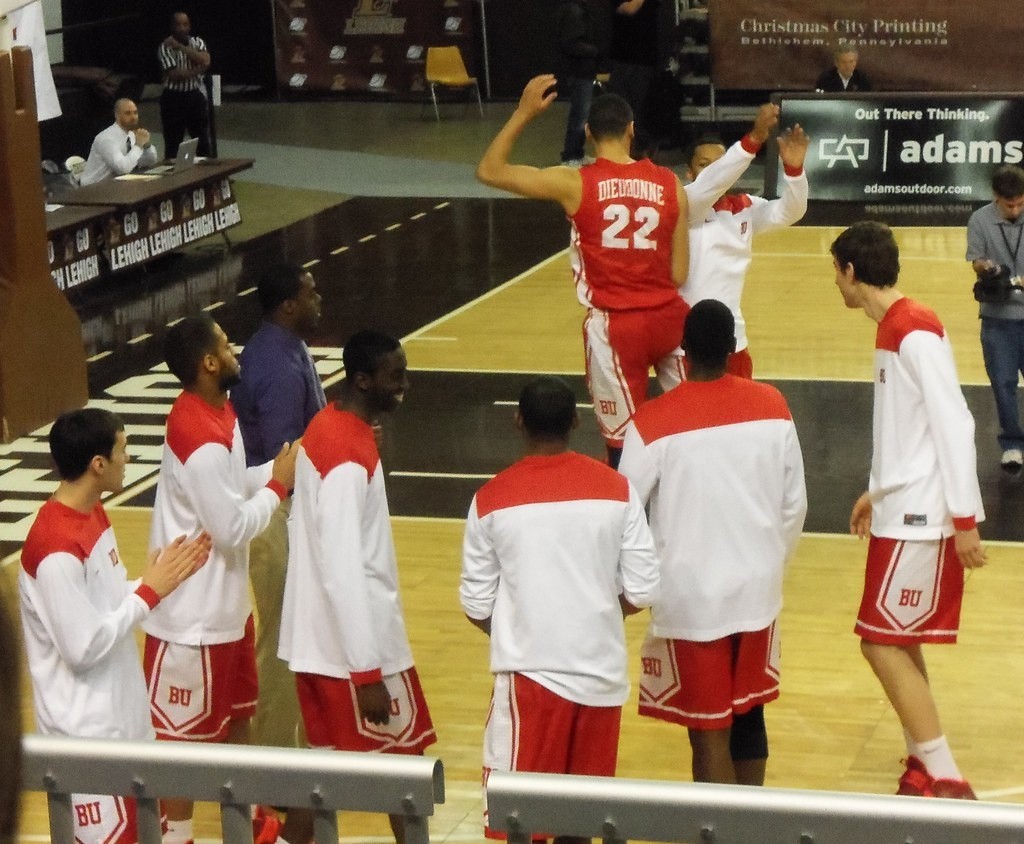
left=1001, top=448, right=1022, bottom=475
left=922, top=779, right=977, bottom=800
left=896, top=753, right=935, bottom=797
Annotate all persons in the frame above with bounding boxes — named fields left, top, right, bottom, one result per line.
left=966, top=165, right=1024, bottom=466
left=459, top=374, right=660, bottom=844
left=678, top=102, right=810, bottom=381
left=277, top=328, right=439, bottom=844
left=560, top=1, right=644, bottom=164
left=816, top=44, right=873, bottom=92
left=830, top=221, right=987, bottom=801
left=227, top=255, right=327, bottom=844
left=141, top=312, right=305, bottom=844
left=77, top=98, right=159, bottom=188
left=617, top=299, right=808, bottom=784
left=17, top=408, right=212, bottom=844
left=475, top=73, right=689, bottom=470
left=160, top=13, right=211, bottom=160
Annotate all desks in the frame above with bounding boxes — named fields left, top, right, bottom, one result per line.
left=44, top=153, right=256, bottom=294
left=764, top=92, right=1024, bottom=227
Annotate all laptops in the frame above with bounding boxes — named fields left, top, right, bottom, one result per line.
left=144, top=137, right=199, bottom=176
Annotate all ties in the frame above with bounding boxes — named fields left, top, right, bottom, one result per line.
left=127, top=137, right=138, bottom=170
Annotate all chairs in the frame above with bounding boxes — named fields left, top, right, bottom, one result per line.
left=420, top=45, right=485, bottom=122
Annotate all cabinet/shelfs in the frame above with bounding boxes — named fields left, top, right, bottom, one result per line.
left=674, top=0, right=716, bottom=123
